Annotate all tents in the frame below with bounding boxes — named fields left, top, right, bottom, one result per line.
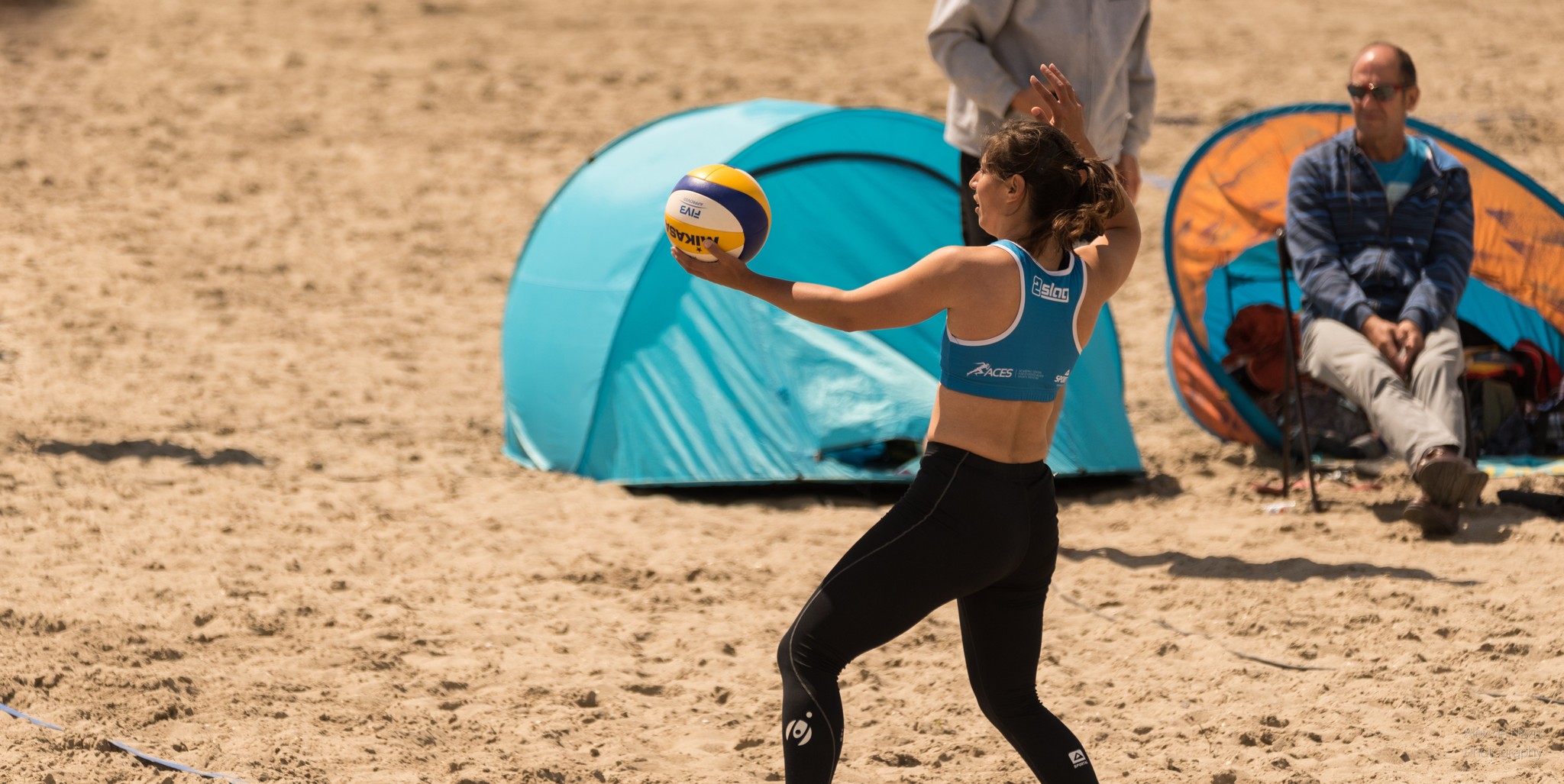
left=503, top=101, right=1149, bottom=489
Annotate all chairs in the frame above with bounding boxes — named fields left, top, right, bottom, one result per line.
left=1270, top=218, right=1490, bottom=515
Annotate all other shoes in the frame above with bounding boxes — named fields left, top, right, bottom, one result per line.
left=1415, top=448, right=1489, bottom=503
left=1404, top=491, right=1463, bottom=535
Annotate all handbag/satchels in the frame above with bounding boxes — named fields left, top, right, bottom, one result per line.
left=1511, top=338, right=1560, bottom=402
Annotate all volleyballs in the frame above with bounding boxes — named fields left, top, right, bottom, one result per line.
left=665, top=165, right=772, bottom=264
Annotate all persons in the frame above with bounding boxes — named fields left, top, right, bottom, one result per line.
left=669, top=64, right=1142, bottom=784
left=925, top=0, right=1155, bottom=252
left=1283, top=41, right=1490, bottom=536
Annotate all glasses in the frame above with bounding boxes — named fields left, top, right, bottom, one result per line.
left=1346, top=83, right=1398, bottom=101
